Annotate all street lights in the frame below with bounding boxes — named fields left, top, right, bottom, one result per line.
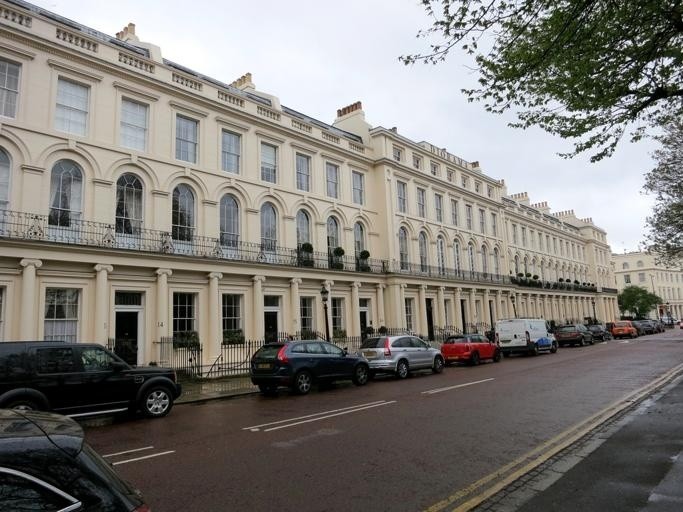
left=320, top=287, right=330, bottom=342
left=510, top=294, right=517, bottom=319
left=591, top=299, right=596, bottom=324
left=665, top=301, right=670, bottom=326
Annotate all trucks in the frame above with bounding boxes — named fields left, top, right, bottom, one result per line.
left=495, top=319, right=559, bottom=358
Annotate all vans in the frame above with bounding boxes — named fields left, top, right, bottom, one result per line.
left=662, top=317, right=681, bottom=325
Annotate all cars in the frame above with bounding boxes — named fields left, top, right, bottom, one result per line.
left=357, top=334, right=444, bottom=378
left=441, top=333, right=501, bottom=366
left=0, top=408, right=151, bottom=512
left=251, top=340, right=369, bottom=395
left=632, top=319, right=665, bottom=336
left=611, top=320, right=638, bottom=339
left=555, top=324, right=612, bottom=347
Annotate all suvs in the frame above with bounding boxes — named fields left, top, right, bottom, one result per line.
left=0, top=341, right=182, bottom=419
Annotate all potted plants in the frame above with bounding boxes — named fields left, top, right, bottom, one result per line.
left=300, top=243, right=370, bottom=272
